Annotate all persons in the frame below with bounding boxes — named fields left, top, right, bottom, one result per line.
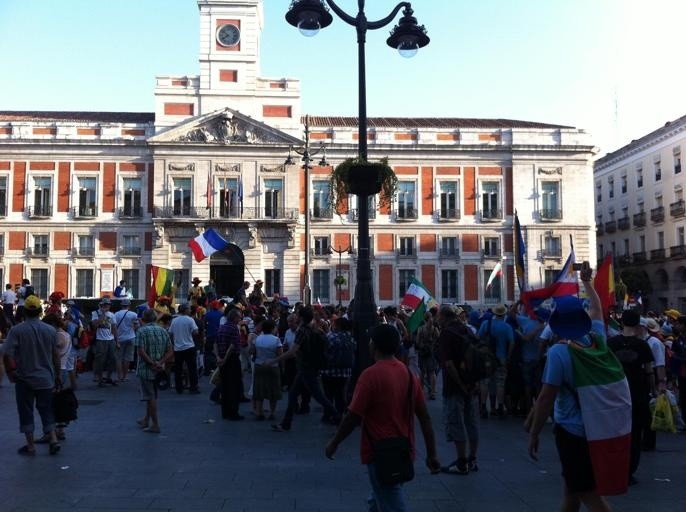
left=324, top=324, right=441, bottom=511
left=1, top=279, right=685, bottom=479
left=528, top=260, right=635, bottom=512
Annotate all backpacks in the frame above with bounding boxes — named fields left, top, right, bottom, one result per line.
left=23, top=286, right=34, bottom=299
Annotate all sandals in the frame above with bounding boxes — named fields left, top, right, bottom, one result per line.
left=50, top=442, right=61, bottom=455
left=18, top=445, right=36, bottom=456
left=94, top=377, right=129, bottom=387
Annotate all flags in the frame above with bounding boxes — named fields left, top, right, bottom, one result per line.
left=592, top=253, right=616, bottom=334
left=513, top=214, right=526, bottom=294
left=518, top=241, right=579, bottom=323
left=188, top=226, right=229, bottom=263
left=148, top=263, right=174, bottom=311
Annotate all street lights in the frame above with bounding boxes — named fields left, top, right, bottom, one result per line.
left=324, top=244, right=356, bottom=306
left=281, top=117, right=329, bottom=307
left=283, top=0, right=431, bottom=414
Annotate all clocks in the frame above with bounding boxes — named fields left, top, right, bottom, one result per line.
left=216, top=22, right=240, bottom=46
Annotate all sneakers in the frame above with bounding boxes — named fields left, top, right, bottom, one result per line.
left=142, top=426, right=161, bottom=433
left=137, top=418, right=150, bottom=428
left=468, top=459, right=480, bottom=472
left=294, top=405, right=352, bottom=425
left=270, top=423, right=291, bottom=432
left=478, top=405, right=529, bottom=418
left=441, top=461, right=469, bottom=475
left=209, top=393, right=280, bottom=421
left=176, top=387, right=202, bottom=394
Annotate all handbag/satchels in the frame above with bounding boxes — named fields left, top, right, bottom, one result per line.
left=370, top=438, right=416, bottom=482
left=52, top=388, right=79, bottom=422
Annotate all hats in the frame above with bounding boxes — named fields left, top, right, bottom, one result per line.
left=120, top=299, right=132, bottom=305
left=46, top=291, right=65, bottom=316
left=640, top=317, right=659, bottom=332
left=256, top=280, right=264, bottom=285
left=664, top=309, right=681, bottom=320
left=191, top=277, right=202, bottom=283
left=276, top=296, right=293, bottom=309
left=492, top=303, right=508, bottom=315
left=99, top=298, right=112, bottom=304
left=549, top=295, right=592, bottom=340
left=24, top=295, right=41, bottom=310
